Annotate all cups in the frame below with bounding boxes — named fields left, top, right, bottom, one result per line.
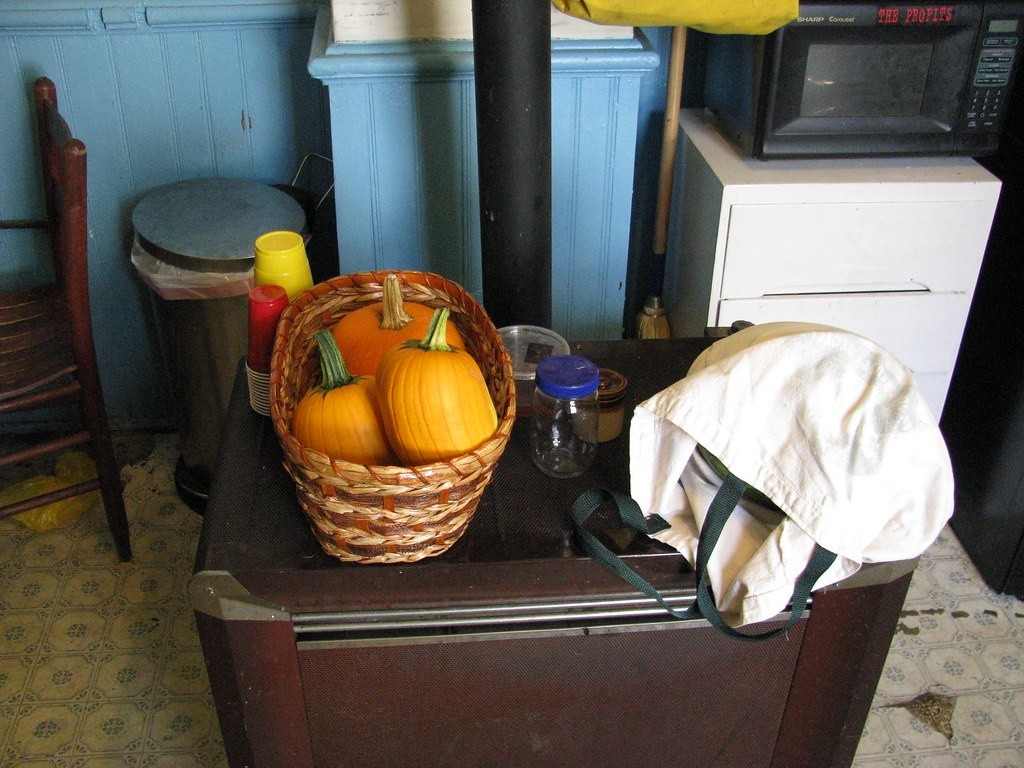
left=246, top=285, right=289, bottom=417
left=255, top=231, right=314, bottom=304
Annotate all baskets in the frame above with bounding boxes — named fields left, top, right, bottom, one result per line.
left=268, top=270, right=517, bottom=564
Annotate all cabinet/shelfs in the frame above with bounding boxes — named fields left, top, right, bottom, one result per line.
left=660, top=107, right=1002, bottom=429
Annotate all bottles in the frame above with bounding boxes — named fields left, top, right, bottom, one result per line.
left=532, top=356, right=600, bottom=478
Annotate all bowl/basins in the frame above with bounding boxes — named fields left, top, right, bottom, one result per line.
left=498, top=325, right=570, bottom=417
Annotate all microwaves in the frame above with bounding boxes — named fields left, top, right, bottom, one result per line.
left=702, top=0, right=1024, bottom=162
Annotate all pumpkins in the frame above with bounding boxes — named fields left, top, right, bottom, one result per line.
left=289, top=328, right=402, bottom=467
left=329, top=274, right=465, bottom=378
left=374, top=307, right=498, bottom=467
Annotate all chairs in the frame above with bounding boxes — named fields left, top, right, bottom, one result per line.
left=1, top=77, right=133, bottom=564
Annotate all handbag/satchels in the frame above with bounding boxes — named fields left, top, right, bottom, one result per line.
left=568, top=322, right=955, bottom=643
